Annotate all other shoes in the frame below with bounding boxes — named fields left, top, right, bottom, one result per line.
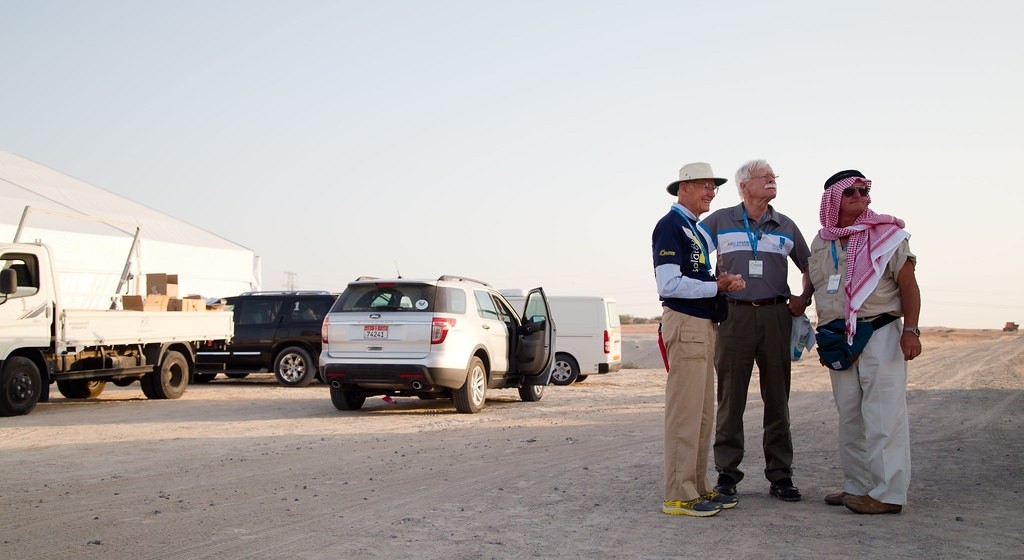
left=841, top=494, right=902, bottom=514
left=825, top=492, right=851, bottom=504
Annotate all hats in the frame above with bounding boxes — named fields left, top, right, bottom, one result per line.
left=666, top=162, right=728, bottom=196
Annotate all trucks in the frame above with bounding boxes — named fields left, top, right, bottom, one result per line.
left=0, top=241, right=237, bottom=418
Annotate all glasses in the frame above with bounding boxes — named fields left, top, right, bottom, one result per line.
left=688, top=181, right=718, bottom=195
left=844, top=187, right=872, bottom=198
left=750, top=174, right=780, bottom=181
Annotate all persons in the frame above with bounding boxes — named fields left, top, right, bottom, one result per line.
left=651, top=162, right=748, bottom=517
left=806, top=169, right=922, bottom=515
left=698, top=159, right=816, bottom=503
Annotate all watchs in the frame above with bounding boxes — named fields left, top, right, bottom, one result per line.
left=902, top=328, right=921, bottom=337
left=800, top=295, right=812, bottom=307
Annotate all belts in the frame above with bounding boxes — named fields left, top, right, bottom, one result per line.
left=857, top=316, right=877, bottom=321
left=725, top=296, right=787, bottom=306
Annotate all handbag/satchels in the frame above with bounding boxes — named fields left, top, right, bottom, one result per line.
left=815, top=313, right=901, bottom=371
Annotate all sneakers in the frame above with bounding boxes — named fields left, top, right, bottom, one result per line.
left=701, top=490, right=738, bottom=509
left=662, top=496, right=723, bottom=517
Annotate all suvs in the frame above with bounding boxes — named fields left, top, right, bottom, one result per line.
left=193, top=289, right=352, bottom=386
left=317, top=274, right=559, bottom=414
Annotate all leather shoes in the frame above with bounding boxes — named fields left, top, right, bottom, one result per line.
left=713, top=484, right=736, bottom=496
left=770, top=478, right=801, bottom=501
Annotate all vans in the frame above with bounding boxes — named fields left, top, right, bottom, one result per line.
left=495, top=288, right=623, bottom=386
